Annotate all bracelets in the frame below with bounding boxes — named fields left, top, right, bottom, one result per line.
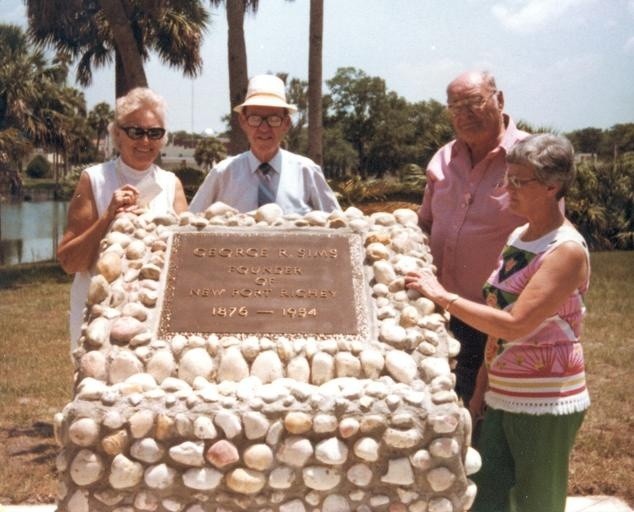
left=445, top=292, right=459, bottom=314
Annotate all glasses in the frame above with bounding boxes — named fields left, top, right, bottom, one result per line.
left=118, top=124, right=166, bottom=139
left=446, top=90, right=496, bottom=117
left=245, top=114, right=285, bottom=127
left=504, top=171, right=536, bottom=188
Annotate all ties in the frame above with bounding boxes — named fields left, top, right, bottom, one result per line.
left=258, top=163, right=275, bottom=206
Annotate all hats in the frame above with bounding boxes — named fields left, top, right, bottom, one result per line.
left=233, top=76, right=297, bottom=114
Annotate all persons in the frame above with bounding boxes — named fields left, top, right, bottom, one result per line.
left=401, top=130, right=595, bottom=511
left=52, top=86, right=186, bottom=385
left=185, top=71, right=344, bottom=214
left=414, top=70, right=566, bottom=445
